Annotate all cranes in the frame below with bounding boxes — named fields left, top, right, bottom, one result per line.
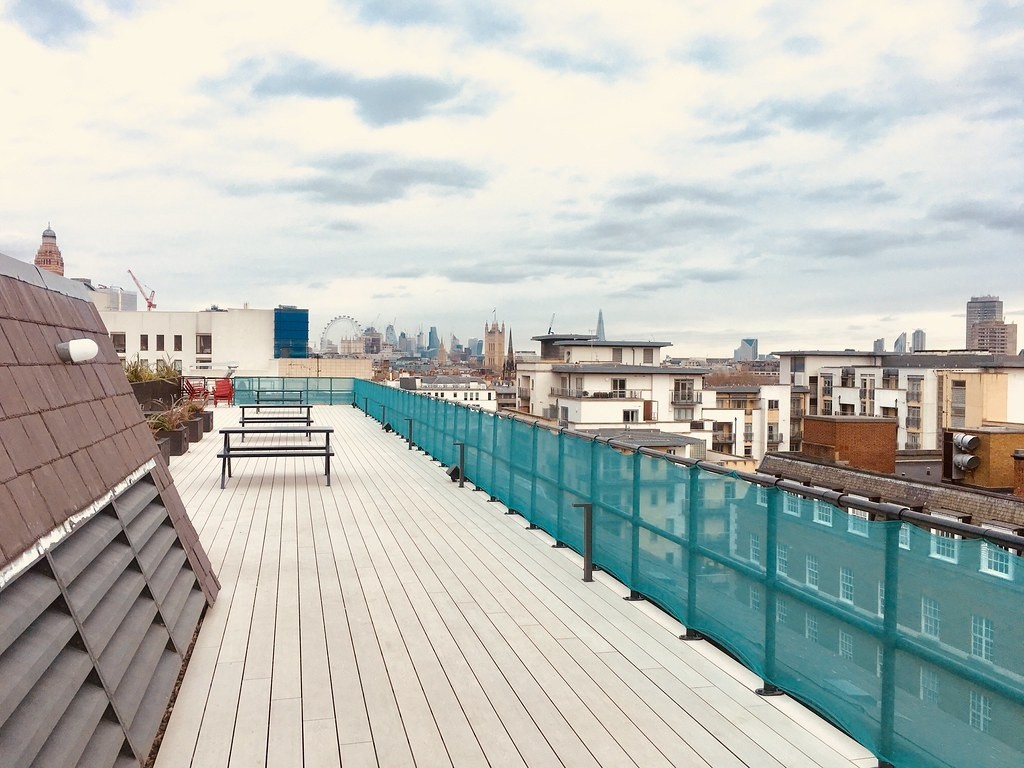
left=127, top=268, right=157, bottom=310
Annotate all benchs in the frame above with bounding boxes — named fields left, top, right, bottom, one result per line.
left=254, top=398, right=303, bottom=414
left=238, top=417, right=314, bottom=442
left=216, top=444, right=335, bottom=487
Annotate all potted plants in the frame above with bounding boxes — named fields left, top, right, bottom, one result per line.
left=143, top=389, right=213, bottom=467
left=582, top=391, right=589, bottom=397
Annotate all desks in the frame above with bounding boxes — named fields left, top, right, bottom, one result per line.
left=255, top=390, right=303, bottom=415
left=219, top=426, right=335, bottom=490
left=239, top=405, right=313, bottom=443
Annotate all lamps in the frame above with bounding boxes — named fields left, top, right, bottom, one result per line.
left=55, top=338, right=99, bottom=363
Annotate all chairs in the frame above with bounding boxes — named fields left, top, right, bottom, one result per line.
left=182, top=377, right=234, bottom=408
